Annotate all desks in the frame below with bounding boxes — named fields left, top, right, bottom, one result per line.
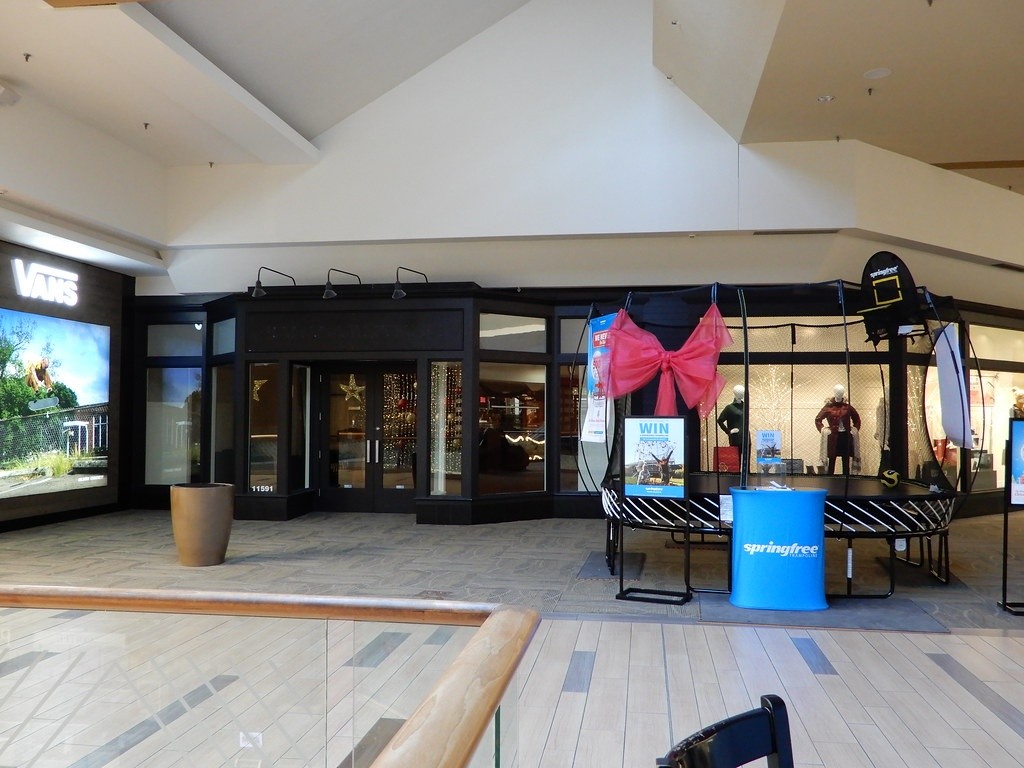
left=730, top=484, right=830, bottom=612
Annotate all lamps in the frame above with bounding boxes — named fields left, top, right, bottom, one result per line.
left=252, top=267, right=298, bottom=298
left=321, top=268, right=362, bottom=299
left=390, top=266, right=428, bottom=300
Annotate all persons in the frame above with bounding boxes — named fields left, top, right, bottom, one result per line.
left=717, top=384, right=752, bottom=477
left=1010, top=385, right=1024, bottom=419
left=875, top=386, right=890, bottom=477
left=815, top=384, right=861, bottom=476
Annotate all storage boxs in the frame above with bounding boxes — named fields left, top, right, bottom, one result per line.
left=170, top=481, right=235, bottom=566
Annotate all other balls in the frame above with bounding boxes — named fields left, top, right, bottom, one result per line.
left=881, top=470, right=901, bottom=489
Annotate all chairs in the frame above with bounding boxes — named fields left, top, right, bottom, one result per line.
left=653, top=693, right=795, bottom=768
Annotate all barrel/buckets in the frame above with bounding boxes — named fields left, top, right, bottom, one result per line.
left=170, top=482, right=235, bottom=567
